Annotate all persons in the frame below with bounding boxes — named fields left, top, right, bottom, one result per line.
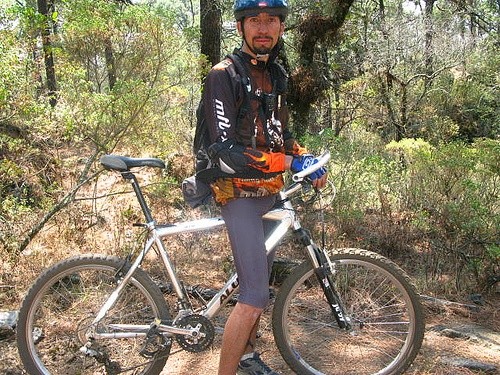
left=201, top=0, right=328, bottom=375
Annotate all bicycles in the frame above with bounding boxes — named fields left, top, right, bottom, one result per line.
left=15, top=149, right=426, bottom=375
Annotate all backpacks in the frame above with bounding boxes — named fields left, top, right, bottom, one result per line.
left=192, top=51, right=291, bottom=185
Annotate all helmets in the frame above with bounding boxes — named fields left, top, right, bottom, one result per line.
left=233, top=0, right=289, bottom=20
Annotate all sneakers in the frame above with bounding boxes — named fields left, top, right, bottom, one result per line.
left=235, top=351, right=280, bottom=375
